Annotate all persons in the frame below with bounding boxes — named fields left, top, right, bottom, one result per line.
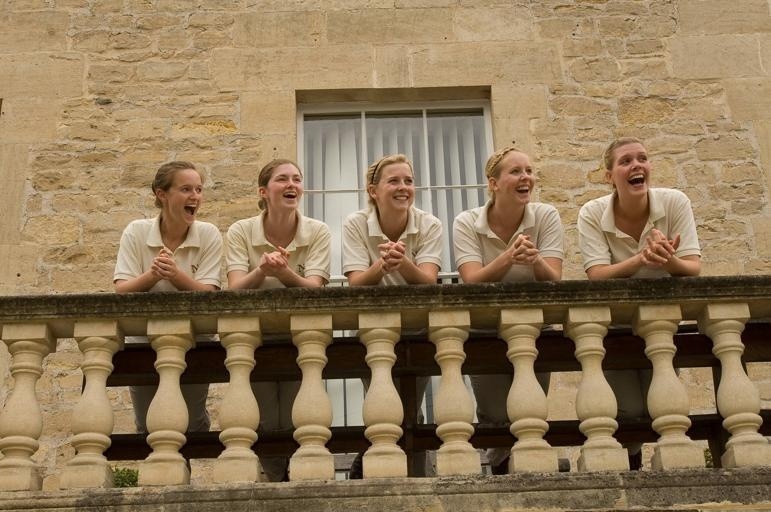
left=223, top=157, right=333, bottom=482
left=576, top=136, right=702, bottom=470
left=113, top=162, right=224, bottom=470
left=452, top=146, right=571, bottom=472
left=339, top=154, right=444, bottom=478
